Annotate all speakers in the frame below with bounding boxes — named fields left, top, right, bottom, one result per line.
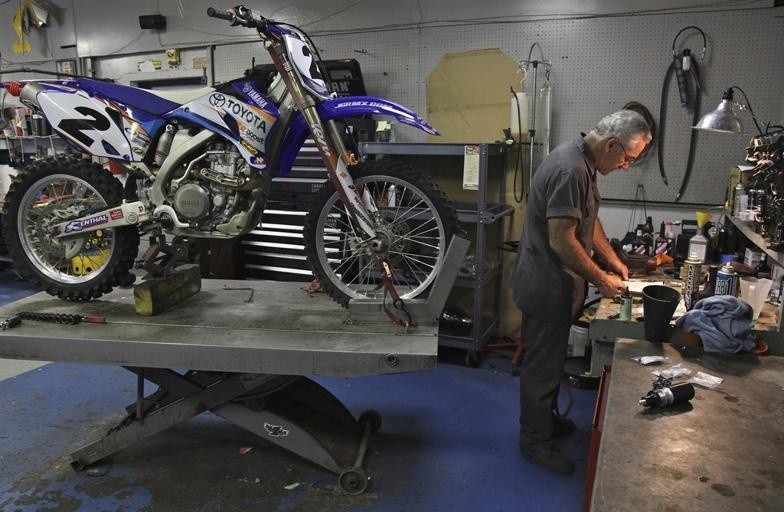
left=138, top=15, right=164, bottom=30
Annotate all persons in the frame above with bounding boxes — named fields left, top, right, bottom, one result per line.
left=509, top=110, right=653, bottom=472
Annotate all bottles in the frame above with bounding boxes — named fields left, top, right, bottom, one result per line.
left=619, top=285, right=634, bottom=322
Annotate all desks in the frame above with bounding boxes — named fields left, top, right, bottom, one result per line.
left=591, top=240, right=784, bottom=357
left=585, top=338, right=784, bottom=511
left=0, top=276, right=440, bottom=494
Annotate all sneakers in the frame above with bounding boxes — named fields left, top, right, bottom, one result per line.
left=523, top=446, right=576, bottom=474
left=552, top=415, right=575, bottom=433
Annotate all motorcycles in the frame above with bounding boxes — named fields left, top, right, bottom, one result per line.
left=2, top=5, right=460, bottom=308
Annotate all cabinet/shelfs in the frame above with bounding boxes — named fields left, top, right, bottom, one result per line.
left=360, top=139, right=515, bottom=356
left=6, top=132, right=59, bottom=162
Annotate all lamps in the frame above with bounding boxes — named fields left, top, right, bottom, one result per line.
left=694, top=85, right=775, bottom=165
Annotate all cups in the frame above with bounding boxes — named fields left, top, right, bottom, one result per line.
left=739, top=275, right=774, bottom=320
left=641, top=283, right=682, bottom=342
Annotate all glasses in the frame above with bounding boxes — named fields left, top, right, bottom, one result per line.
left=610, top=136, right=636, bottom=164
